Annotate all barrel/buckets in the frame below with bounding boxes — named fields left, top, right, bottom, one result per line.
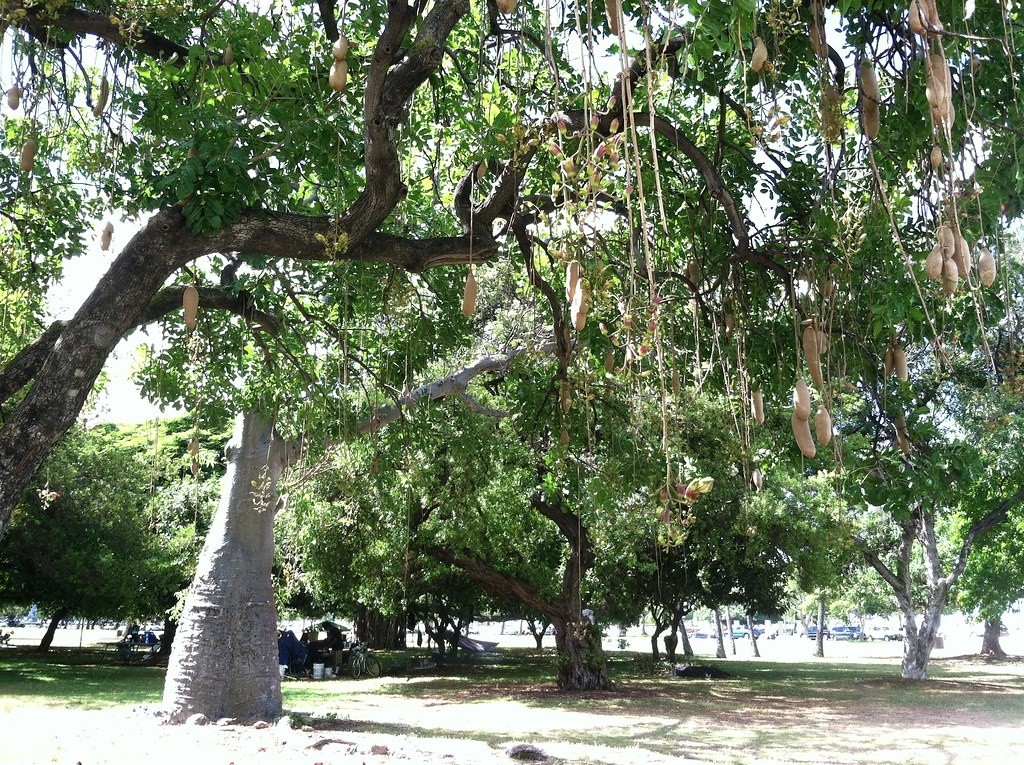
left=279, top=664, right=285, bottom=680
left=325, top=668, right=332, bottom=678
left=313, top=663, right=324, bottom=679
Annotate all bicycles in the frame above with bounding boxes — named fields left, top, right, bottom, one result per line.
left=348, top=635, right=381, bottom=680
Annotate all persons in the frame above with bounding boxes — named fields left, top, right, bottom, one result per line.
left=322, top=621, right=343, bottom=652
left=132, top=622, right=139, bottom=643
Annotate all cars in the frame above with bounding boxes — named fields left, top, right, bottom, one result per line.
left=863, top=625, right=898, bottom=642
left=721, top=629, right=752, bottom=640
left=807, top=625, right=830, bottom=641
left=831, top=626, right=860, bottom=642
